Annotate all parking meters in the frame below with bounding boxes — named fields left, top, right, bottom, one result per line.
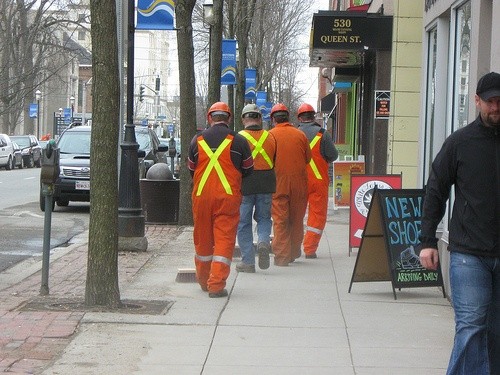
left=38, top=139, right=61, bottom=296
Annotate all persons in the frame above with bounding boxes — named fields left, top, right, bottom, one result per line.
left=187, top=102, right=253, bottom=297
left=295, top=102, right=338, bottom=258
left=418, top=72, right=500, bottom=375
left=237, top=104, right=278, bottom=273
left=267, top=102, right=312, bottom=267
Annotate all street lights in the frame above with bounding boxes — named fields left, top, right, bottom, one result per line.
left=70, top=95, right=75, bottom=123
left=58, top=107, right=63, bottom=138
left=35, top=89, right=42, bottom=140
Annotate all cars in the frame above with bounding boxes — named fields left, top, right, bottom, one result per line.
left=124, top=125, right=182, bottom=179
left=38, top=141, right=56, bottom=168
left=0, top=134, right=14, bottom=170
left=11, top=140, right=24, bottom=169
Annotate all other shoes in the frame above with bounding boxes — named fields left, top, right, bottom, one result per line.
left=208, top=288, right=228, bottom=298
left=258, top=242, right=270, bottom=270
left=305, top=253, right=317, bottom=258
left=236, top=262, right=255, bottom=273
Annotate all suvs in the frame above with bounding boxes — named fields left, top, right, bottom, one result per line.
left=37, top=121, right=91, bottom=213
left=9, top=135, right=43, bottom=168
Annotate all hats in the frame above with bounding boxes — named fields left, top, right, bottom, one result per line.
left=476, top=72, right=500, bottom=101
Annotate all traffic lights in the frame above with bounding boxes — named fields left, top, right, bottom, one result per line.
left=140, top=87, right=145, bottom=102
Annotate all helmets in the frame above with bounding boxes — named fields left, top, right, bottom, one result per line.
left=297, top=103, right=315, bottom=119
left=207, top=101, right=232, bottom=118
left=241, top=103, right=261, bottom=118
left=270, top=103, right=289, bottom=118
